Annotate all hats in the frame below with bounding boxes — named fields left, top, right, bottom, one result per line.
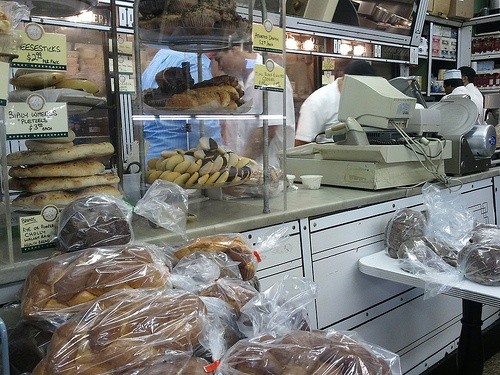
left=443, top=69, right=462, bottom=80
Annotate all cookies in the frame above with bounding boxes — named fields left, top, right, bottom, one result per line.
left=146, top=137, right=279, bottom=188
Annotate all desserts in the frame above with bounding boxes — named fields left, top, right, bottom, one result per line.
left=138, top=0, right=252, bottom=40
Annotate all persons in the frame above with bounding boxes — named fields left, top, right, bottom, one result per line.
left=141, top=48, right=224, bottom=163
left=213, top=42, right=296, bottom=166
left=440, top=69, right=478, bottom=107
left=294, top=58, right=377, bottom=145
left=460, top=66, right=483, bottom=121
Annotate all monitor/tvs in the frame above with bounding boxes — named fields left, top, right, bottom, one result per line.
left=338, top=74, right=417, bottom=130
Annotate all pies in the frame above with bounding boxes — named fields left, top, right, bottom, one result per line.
left=7, top=129, right=124, bottom=208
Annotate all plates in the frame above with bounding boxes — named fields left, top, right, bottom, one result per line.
left=140, top=27, right=251, bottom=51
left=179, top=180, right=247, bottom=189
left=30, top=0, right=99, bottom=19
left=57, top=94, right=108, bottom=115
left=142, top=106, right=252, bottom=114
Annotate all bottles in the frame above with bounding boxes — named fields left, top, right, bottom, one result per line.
left=471, top=37, right=500, bottom=54
left=474, top=73, right=500, bottom=87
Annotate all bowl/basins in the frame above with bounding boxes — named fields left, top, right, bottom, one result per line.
left=287, top=174, right=296, bottom=186
left=300, top=174, right=323, bottom=190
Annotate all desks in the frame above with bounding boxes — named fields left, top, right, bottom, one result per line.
left=357, top=239, right=500, bottom=375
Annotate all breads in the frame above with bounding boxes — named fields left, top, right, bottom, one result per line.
left=58, top=197, right=131, bottom=252
left=142, top=66, right=246, bottom=109
left=19, top=237, right=393, bottom=375
left=384, top=209, right=500, bottom=287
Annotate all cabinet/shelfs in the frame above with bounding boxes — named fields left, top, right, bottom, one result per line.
left=0, top=0, right=500, bottom=375
